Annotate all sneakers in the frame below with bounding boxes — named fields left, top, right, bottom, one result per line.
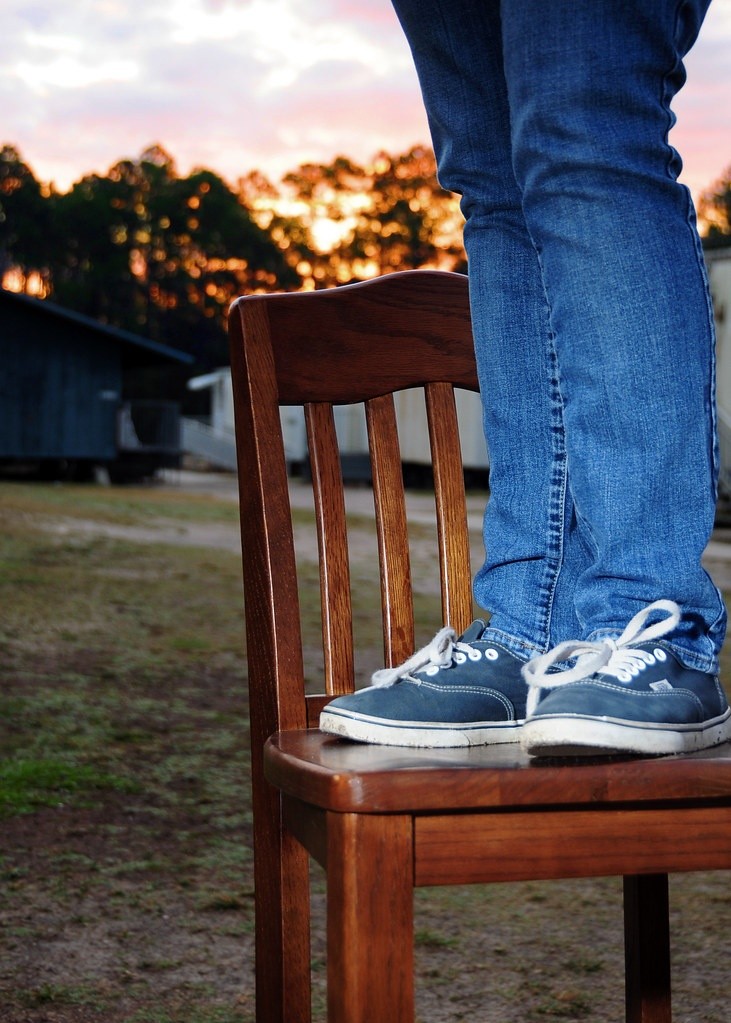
left=520, top=600, right=731, bottom=752
left=318, top=621, right=559, bottom=748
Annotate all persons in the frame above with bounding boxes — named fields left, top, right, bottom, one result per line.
left=317, top=0, right=729, bottom=756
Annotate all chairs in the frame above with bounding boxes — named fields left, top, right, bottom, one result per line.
left=228, top=269, right=731, bottom=1022
